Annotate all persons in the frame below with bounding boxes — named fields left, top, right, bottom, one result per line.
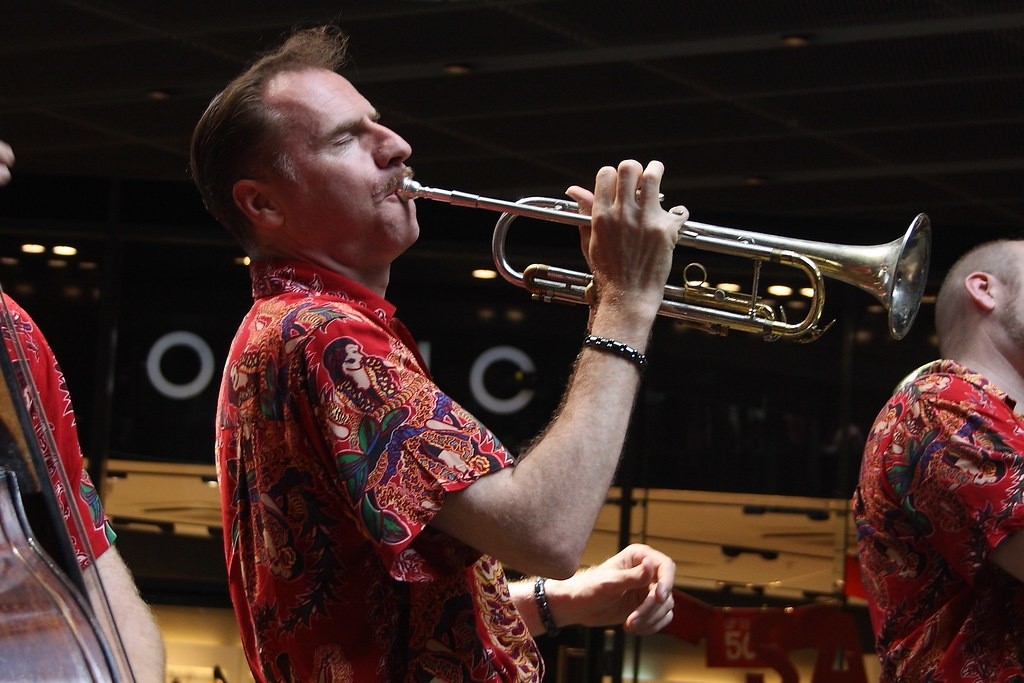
left=191, top=35, right=689, bottom=683
left=0, top=139, right=170, bottom=683
left=852, top=239, right=1024, bottom=683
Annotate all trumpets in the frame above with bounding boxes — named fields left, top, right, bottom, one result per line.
left=396, top=174, right=935, bottom=347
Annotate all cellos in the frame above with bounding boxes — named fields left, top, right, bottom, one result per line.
left=0, top=293, right=135, bottom=683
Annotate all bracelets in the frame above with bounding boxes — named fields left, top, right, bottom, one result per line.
left=583, top=332, right=649, bottom=371
left=534, top=576, right=560, bottom=639
